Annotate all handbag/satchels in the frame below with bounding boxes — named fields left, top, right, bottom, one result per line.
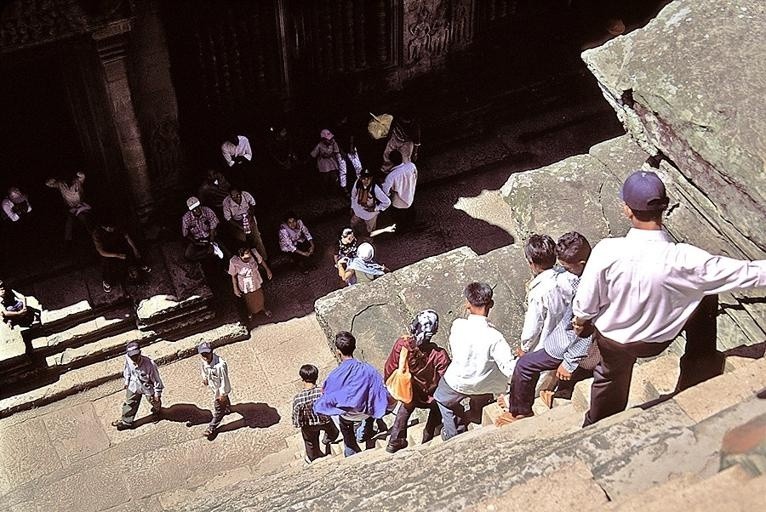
left=385, top=348, right=414, bottom=404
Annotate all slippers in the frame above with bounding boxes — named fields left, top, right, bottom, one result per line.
left=495, top=412, right=516, bottom=428
left=540, top=389, right=554, bottom=408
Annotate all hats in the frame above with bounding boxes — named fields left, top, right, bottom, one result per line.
left=126, top=342, right=140, bottom=357
left=359, top=167, right=372, bottom=178
left=621, top=170, right=668, bottom=212
left=198, top=341, right=215, bottom=354
left=320, top=128, right=335, bottom=140
left=186, top=196, right=201, bottom=211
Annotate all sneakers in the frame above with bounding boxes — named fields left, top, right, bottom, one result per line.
left=211, top=241, right=224, bottom=259
left=102, top=280, right=112, bottom=293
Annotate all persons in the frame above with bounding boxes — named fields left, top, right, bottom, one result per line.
left=198, top=342, right=231, bottom=435
left=1, top=173, right=152, bottom=327
left=293, top=231, right=600, bottom=462
left=112, top=343, right=163, bottom=428
left=570, top=171, right=766, bottom=428
left=182, top=116, right=419, bottom=321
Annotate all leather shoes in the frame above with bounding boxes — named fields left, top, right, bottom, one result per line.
left=385, top=439, right=408, bottom=453
left=111, top=419, right=131, bottom=429
left=203, top=427, right=215, bottom=437
left=153, top=409, right=160, bottom=424
